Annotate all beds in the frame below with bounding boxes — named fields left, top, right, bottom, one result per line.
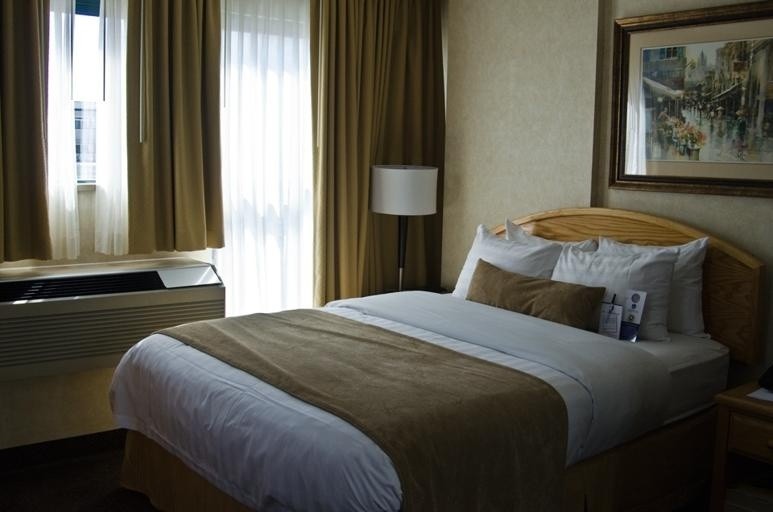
left=106, top=205, right=762, bottom=512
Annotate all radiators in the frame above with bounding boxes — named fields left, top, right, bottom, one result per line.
left=0, top=255, right=225, bottom=470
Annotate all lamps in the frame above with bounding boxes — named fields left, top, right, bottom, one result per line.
left=368, top=164, right=438, bottom=291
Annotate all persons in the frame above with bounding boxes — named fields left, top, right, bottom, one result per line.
left=657, top=107, right=672, bottom=122
left=735, top=110, right=747, bottom=160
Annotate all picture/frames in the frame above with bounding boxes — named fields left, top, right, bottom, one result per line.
left=608, top=0, right=773, bottom=198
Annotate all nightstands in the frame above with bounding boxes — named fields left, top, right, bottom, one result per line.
left=713, top=372, right=773, bottom=512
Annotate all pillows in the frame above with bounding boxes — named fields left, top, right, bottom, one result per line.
left=451, top=218, right=710, bottom=341
